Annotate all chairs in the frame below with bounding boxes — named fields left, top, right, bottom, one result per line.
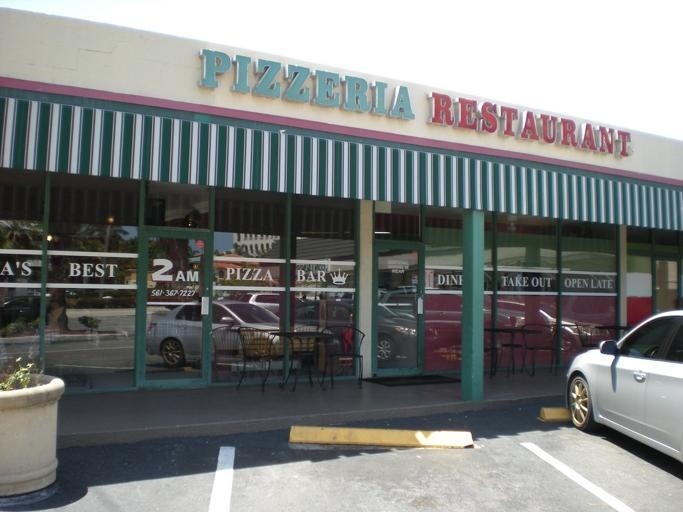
left=485, top=323, right=557, bottom=380
left=210, top=325, right=251, bottom=381
left=236, top=324, right=366, bottom=392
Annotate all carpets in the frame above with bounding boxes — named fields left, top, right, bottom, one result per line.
left=360, top=375, right=460, bottom=387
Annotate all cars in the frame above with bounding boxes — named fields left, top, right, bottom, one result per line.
left=564, top=308, right=683, bottom=465
left=0, top=294, right=59, bottom=330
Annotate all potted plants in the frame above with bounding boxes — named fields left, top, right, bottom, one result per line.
left=0, top=356, right=65, bottom=498
